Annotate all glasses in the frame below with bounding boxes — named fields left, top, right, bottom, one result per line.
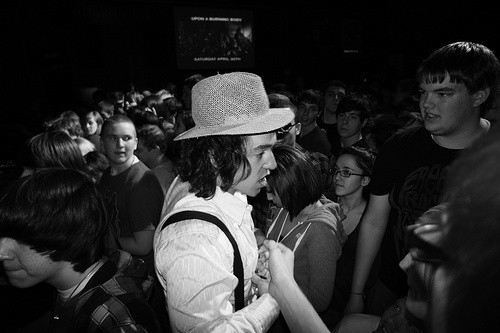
left=329, top=167, right=362, bottom=179
left=277, top=121, right=297, bottom=140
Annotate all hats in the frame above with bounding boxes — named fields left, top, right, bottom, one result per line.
left=173, top=71, right=296, bottom=141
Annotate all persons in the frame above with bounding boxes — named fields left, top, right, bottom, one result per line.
left=0, top=25, right=500, bottom=333
